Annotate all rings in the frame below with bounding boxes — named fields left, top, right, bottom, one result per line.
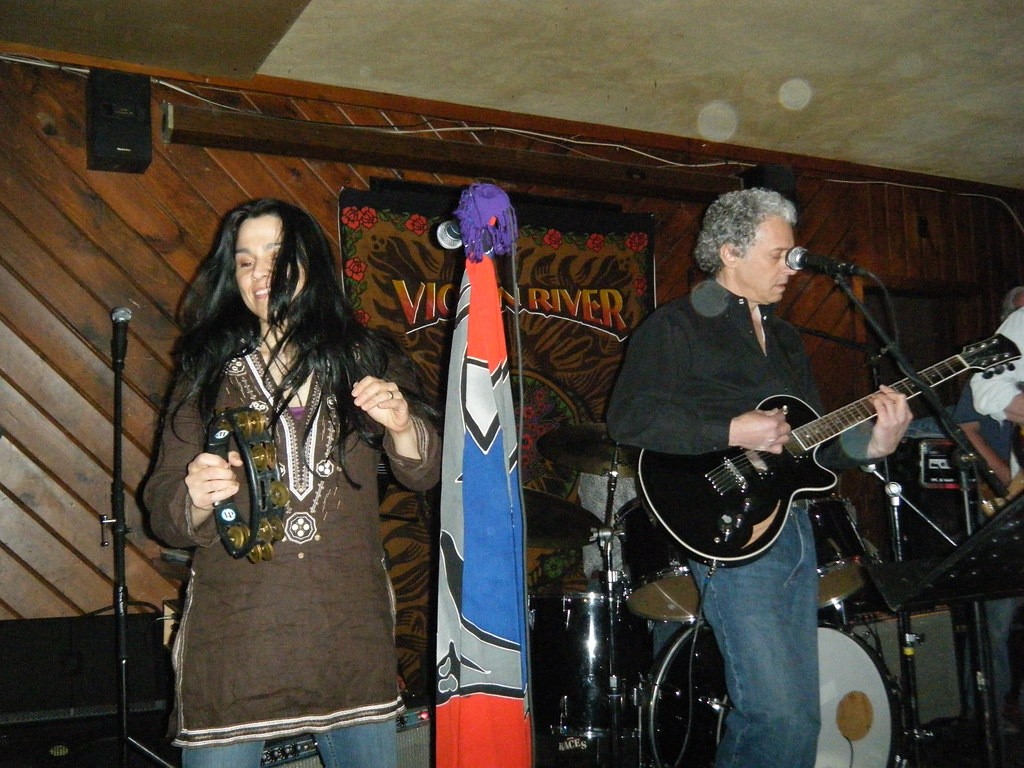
left=388, top=391, right=393, bottom=399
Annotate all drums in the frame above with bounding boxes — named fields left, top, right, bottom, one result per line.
left=526, top=590, right=656, bottom=757
left=805, top=495, right=871, bottom=611
left=642, top=618, right=910, bottom=768
left=612, top=494, right=708, bottom=624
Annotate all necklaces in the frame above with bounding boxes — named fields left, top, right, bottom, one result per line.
left=260, top=335, right=302, bottom=407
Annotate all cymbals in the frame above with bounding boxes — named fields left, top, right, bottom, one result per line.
left=536, top=421, right=641, bottom=480
left=204, top=405, right=292, bottom=565
left=519, top=485, right=606, bottom=551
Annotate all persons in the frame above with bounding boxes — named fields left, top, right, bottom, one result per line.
left=951, top=287, right=1024, bottom=484
left=142, top=199, right=443, bottom=768
left=607, top=190, right=915, bottom=767
left=578, top=473, right=636, bottom=578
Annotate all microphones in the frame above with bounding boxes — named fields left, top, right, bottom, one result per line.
left=111, top=306, right=132, bottom=371
left=786, top=244, right=868, bottom=276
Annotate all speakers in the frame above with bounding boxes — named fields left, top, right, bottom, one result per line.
left=84, top=69, right=152, bottom=174
left=0, top=613, right=176, bottom=768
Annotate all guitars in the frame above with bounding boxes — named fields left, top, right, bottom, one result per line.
left=632, top=333, right=1022, bottom=570
